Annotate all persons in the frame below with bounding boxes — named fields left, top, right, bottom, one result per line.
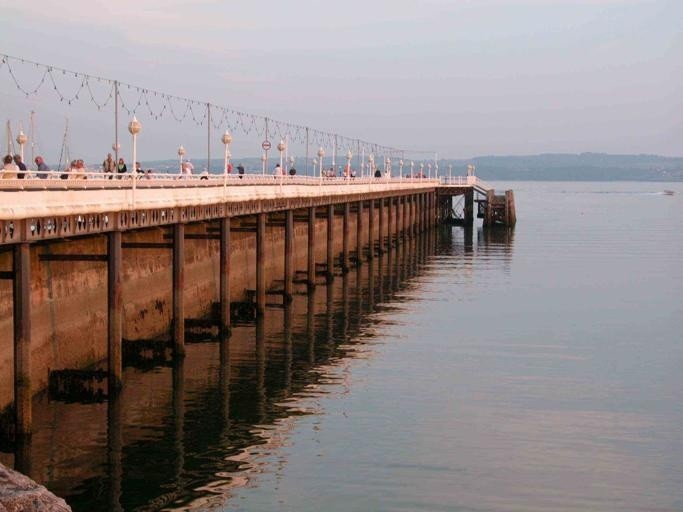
left=322, top=166, right=356, bottom=180
left=136, top=162, right=153, bottom=179
left=236, top=162, right=245, bottom=179
left=273, top=164, right=296, bottom=179
left=102, top=153, right=116, bottom=179
left=64, top=160, right=78, bottom=179
left=199, top=168, right=208, bottom=179
left=35, top=156, right=50, bottom=179
left=12, top=153, right=26, bottom=179
left=228, top=161, right=233, bottom=173
left=118, top=159, right=127, bottom=179
left=76, top=159, right=88, bottom=180
left=182, top=159, right=193, bottom=179
left=375, top=167, right=381, bottom=177
left=5, top=155, right=21, bottom=179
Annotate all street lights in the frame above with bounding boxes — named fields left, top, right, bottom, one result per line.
left=128, top=114, right=141, bottom=211
left=346, top=150, right=352, bottom=180
left=317, top=147, right=325, bottom=185
left=278, top=139, right=286, bottom=186
left=261, top=154, right=267, bottom=175
left=385, top=158, right=438, bottom=184
left=112, top=142, right=120, bottom=175
left=178, top=143, right=186, bottom=174
left=16, top=131, right=27, bottom=164
left=221, top=130, right=233, bottom=200
left=368, top=155, right=374, bottom=177
left=311, top=159, right=316, bottom=176
